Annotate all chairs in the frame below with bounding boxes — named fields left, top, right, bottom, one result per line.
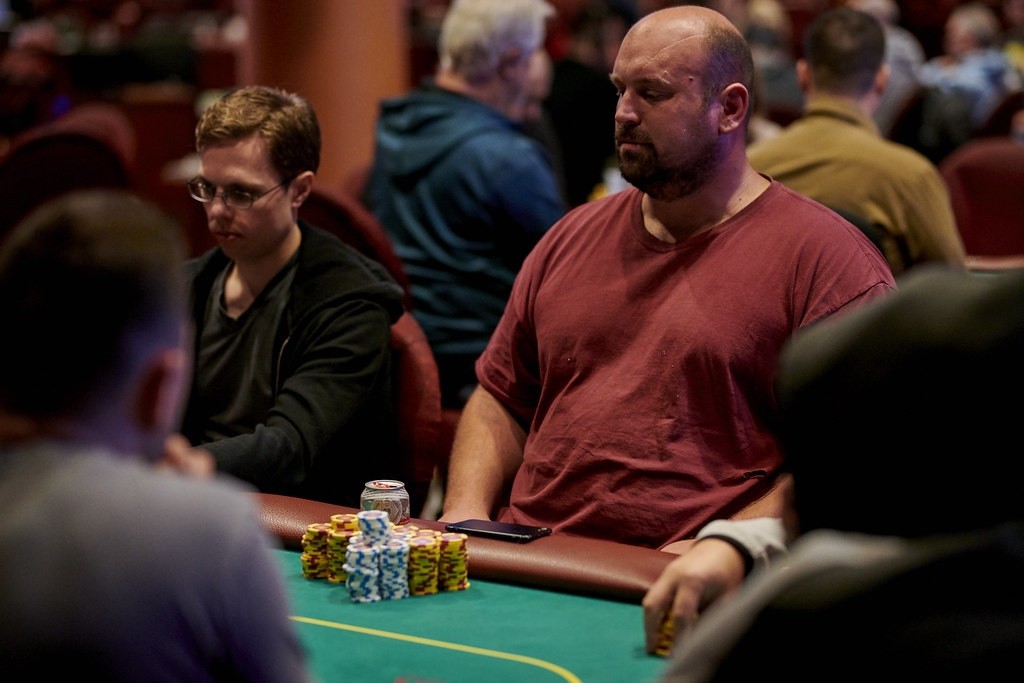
left=292, top=186, right=406, bottom=291
left=391, top=312, right=442, bottom=504
left=934, top=135, right=1024, bottom=261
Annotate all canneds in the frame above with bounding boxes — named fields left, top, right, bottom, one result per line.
left=360, top=480, right=410, bottom=528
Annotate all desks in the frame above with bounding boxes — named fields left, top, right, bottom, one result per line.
left=248, top=490, right=685, bottom=683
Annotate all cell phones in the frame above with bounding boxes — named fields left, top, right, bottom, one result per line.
left=445, top=518, right=552, bottom=543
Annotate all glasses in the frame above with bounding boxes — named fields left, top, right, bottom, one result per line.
left=186, top=175, right=284, bottom=211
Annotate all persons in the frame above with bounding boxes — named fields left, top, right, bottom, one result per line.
left=0, top=189, right=310, bottom=683
left=846, top=0, right=923, bottom=96
left=161, top=86, right=406, bottom=510
left=359, top=0, right=571, bottom=412
left=435, top=5, right=899, bottom=561
left=641, top=517, right=786, bottom=653
left=510, top=0, right=640, bottom=210
left=921, top=2, right=1021, bottom=121
left=728, top=0, right=802, bottom=107
left=745, top=8, right=969, bottom=285
